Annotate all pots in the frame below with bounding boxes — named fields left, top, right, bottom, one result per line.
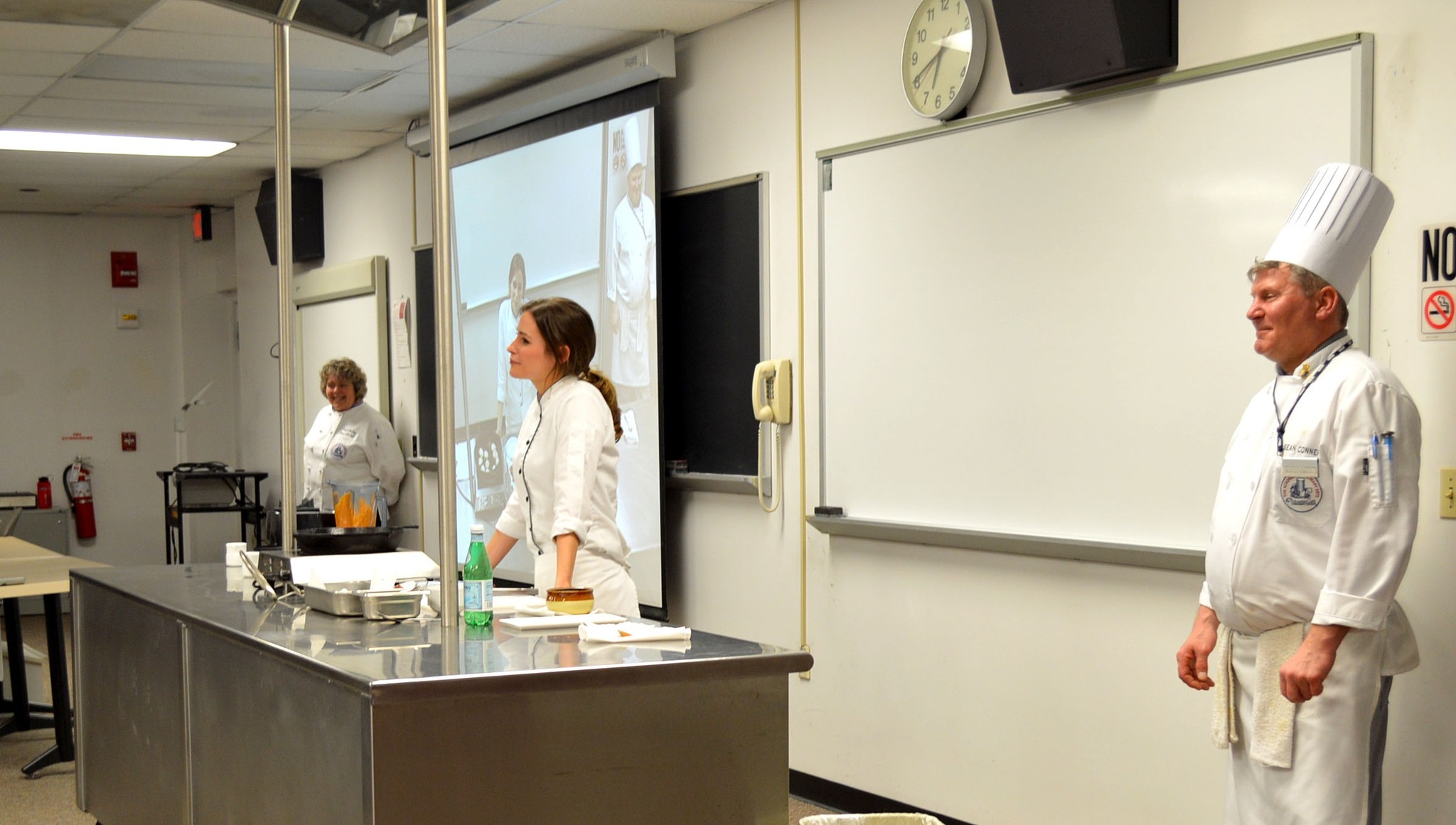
left=292, top=524, right=419, bottom=554
left=265, top=501, right=335, bottom=545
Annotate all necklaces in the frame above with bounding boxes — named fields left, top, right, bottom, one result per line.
left=627, top=192, right=647, bottom=240
left=1272, top=341, right=1355, bottom=455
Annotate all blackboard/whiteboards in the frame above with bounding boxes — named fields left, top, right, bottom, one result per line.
left=814, top=33, right=1372, bottom=580
left=407, top=172, right=770, bottom=498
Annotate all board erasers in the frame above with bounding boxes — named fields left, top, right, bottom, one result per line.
left=815, top=505, right=842, bottom=517
left=665, top=460, right=688, bottom=475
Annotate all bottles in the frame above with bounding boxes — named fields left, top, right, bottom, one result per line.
left=462, top=524, right=494, bottom=627
left=37, top=476, right=52, bottom=509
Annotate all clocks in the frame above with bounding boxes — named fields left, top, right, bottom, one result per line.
left=900, top=0, right=987, bottom=120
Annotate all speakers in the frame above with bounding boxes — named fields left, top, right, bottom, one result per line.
left=254, top=175, right=324, bottom=265
left=990, top=0, right=1179, bottom=95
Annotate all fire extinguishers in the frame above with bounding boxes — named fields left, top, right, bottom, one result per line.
left=63, top=456, right=98, bottom=541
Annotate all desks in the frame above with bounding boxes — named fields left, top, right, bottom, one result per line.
left=0, top=534, right=114, bottom=778
left=154, top=471, right=268, bottom=564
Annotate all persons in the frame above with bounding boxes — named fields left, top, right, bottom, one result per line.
left=496, top=253, right=542, bottom=438
left=482, top=297, right=643, bottom=623
left=301, top=359, right=406, bottom=531
left=1177, top=164, right=1421, bottom=825
left=608, top=114, right=655, bottom=404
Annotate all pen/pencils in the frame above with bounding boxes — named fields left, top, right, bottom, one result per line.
left=376, top=429, right=380, bottom=439
left=1373, top=430, right=1380, bottom=459
left=1381, top=430, right=1394, bottom=460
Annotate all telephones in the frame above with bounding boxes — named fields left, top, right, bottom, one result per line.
left=752, top=359, right=792, bottom=426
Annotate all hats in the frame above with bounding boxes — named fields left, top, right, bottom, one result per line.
left=1259, top=162, right=1395, bottom=307
left=620, top=117, right=645, bottom=178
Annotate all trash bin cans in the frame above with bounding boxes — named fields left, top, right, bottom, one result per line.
left=799, top=812, right=945, bottom=825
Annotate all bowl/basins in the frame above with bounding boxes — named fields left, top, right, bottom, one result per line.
left=351, top=590, right=431, bottom=621
left=323, top=579, right=371, bottom=592
left=547, top=587, right=594, bottom=614
left=428, top=580, right=465, bottom=618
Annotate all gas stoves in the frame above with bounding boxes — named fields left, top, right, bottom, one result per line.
left=252, top=547, right=442, bottom=598
